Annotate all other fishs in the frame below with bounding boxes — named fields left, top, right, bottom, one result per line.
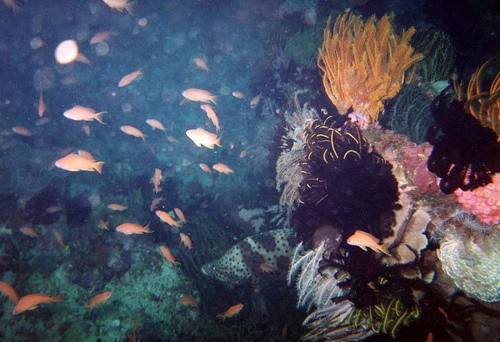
left=0, top=0, right=392, bottom=323
